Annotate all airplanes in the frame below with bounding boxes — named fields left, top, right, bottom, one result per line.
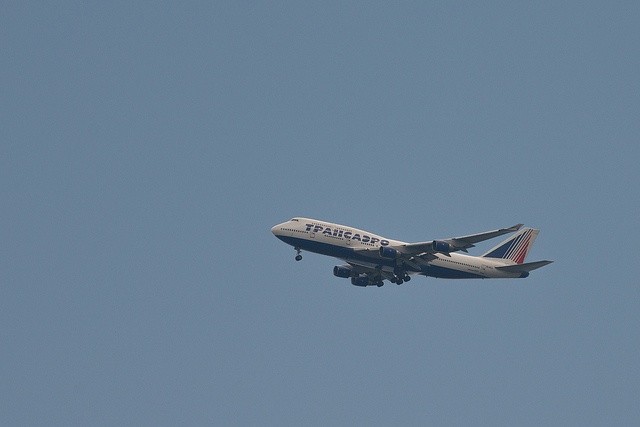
left=270, top=216, right=554, bottom=289
